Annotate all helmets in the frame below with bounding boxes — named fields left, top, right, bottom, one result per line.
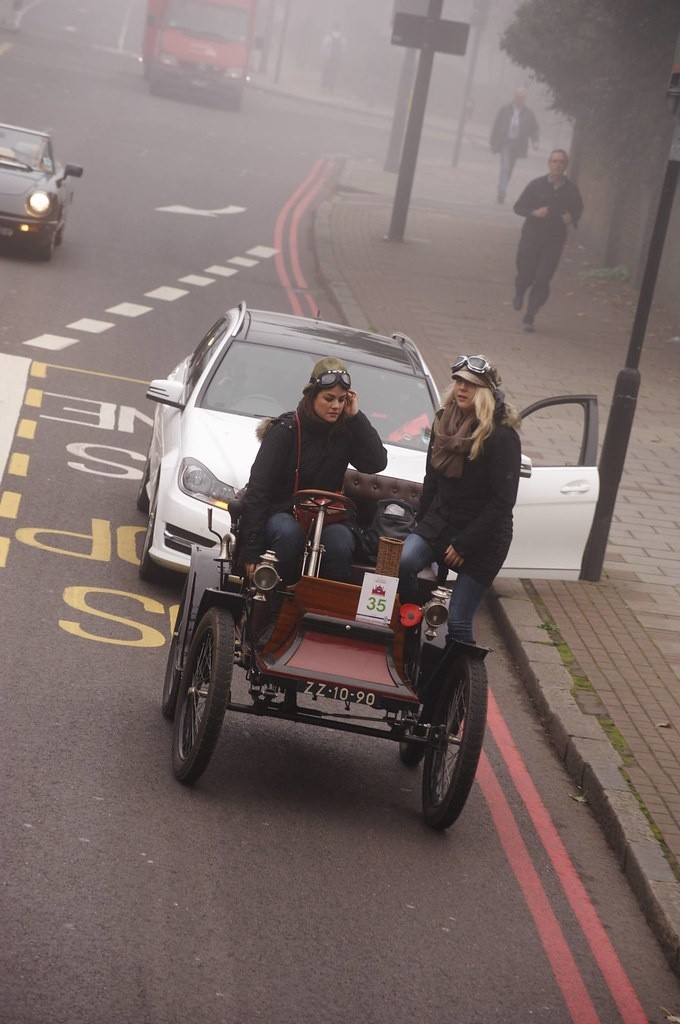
left=303, top=356, right=349, bottom=393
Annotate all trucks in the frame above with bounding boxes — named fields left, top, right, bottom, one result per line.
left=144, top=0, right=257, bottom=109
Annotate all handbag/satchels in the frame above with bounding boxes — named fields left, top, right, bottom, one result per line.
left=294, top=490, right=350, bottom=531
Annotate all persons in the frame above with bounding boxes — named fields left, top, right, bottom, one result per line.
left=318, top=23, right=348, bottom=98
left=489, top=85, right=540, bottom=202
left=512, top=150, right=583, bottom=333
left=399, top=354, right=522, bottom=670
left=238, top=357, right=388, bottom=627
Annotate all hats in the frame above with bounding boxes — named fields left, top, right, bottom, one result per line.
left=452, top=354, right=504, bottom=393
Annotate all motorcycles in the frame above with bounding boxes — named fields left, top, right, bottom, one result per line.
left=153, top=477, right=491, bottom=834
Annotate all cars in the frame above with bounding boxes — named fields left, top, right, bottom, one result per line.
left=0, top=124, right=84, bottom=263
left=133, top=301, right=604, bottom=622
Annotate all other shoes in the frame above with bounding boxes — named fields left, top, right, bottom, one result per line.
left=497, top=192, right=506, bottom=204
left=523, top=321, right=535, bottom=332
left=513, top=294, right=522, bottom=312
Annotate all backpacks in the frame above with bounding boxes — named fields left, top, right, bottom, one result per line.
left=359, top=496, right=417, bottom=563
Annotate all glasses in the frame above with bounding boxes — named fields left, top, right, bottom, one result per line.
left=452, top=356, right=498, bottom=386
left=310, top=370, right=352, bottom=390
